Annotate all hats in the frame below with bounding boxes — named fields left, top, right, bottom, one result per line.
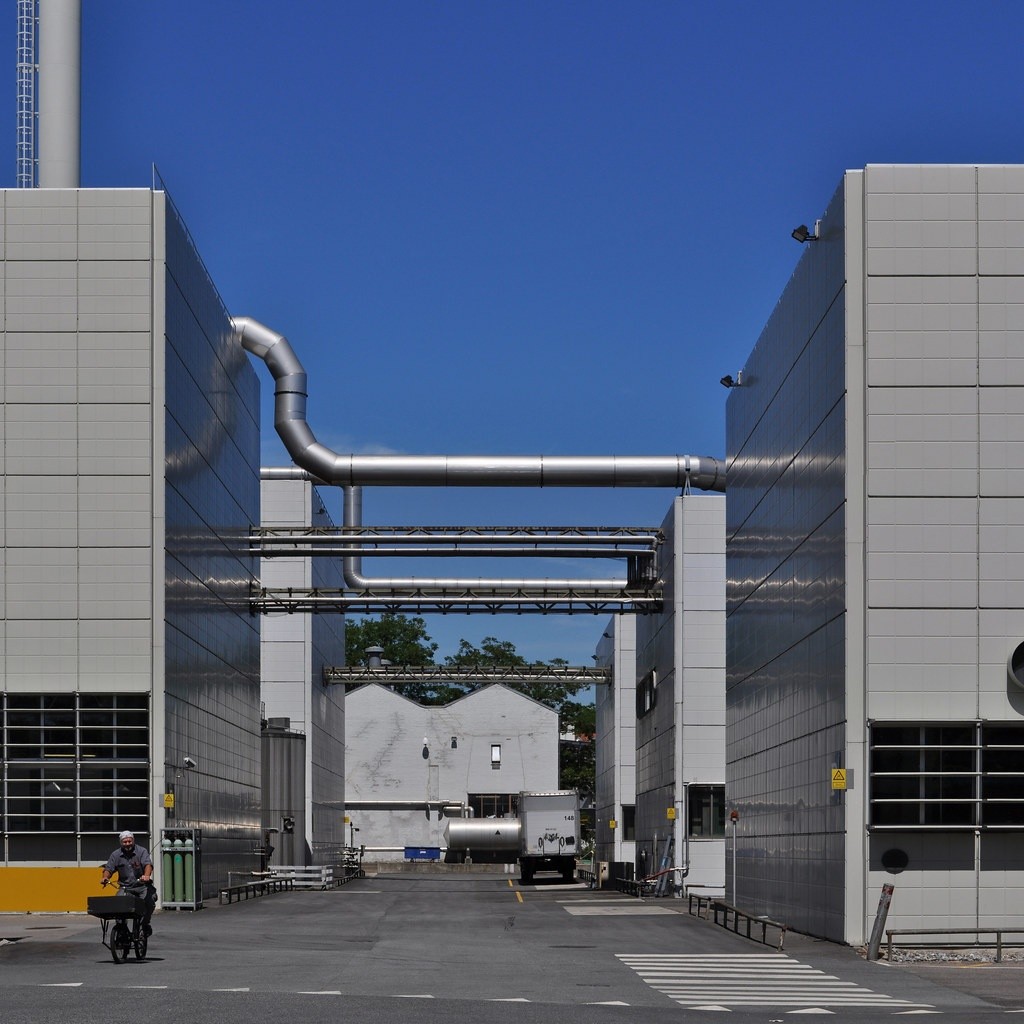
left=119, top=831, right=134, bottom=841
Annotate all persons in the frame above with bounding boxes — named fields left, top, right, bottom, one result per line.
left=100, top=829, right=158, bottom=951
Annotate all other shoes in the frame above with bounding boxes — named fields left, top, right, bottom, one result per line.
left=146, top=925, right=152, bottom=934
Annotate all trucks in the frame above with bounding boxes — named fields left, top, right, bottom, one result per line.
left=519, top=787, right=582, bottom=883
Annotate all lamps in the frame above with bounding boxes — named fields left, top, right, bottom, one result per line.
left=719, top=375, right=741, bottom=390
left=591, top=655, right=600, bottom=661
left=423, top=737, right=428, bottom=751
left=602, top=632, right=612, bottom=638
left=792, top=225, right=819, bottom=245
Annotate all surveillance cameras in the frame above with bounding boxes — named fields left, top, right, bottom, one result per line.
left=183, top=757, right=197, bottom=767
left=423, top=738, right=428, bottom=745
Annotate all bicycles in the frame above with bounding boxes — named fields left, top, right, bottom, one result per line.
left=87, top=879, right=153, bottom=964
